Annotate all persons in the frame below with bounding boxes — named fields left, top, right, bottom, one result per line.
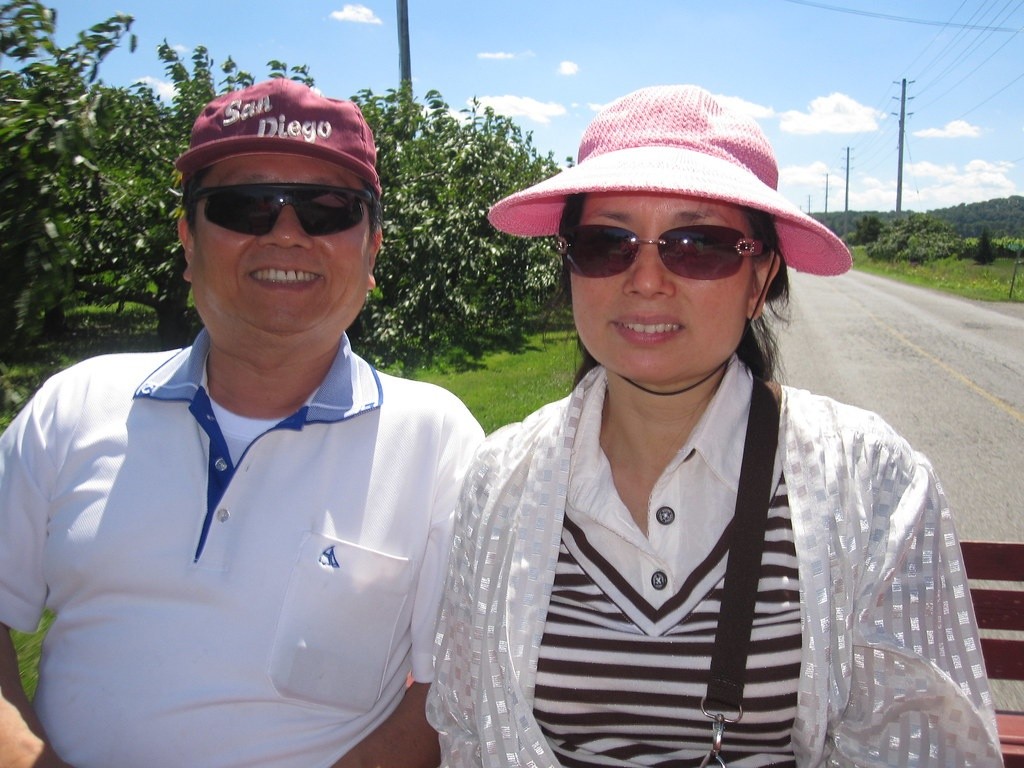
left=424, top=84, right=1009, bottom=768
left=0, top=79, right=488, bottom=768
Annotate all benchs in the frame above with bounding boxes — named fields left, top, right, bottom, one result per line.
left=957, top=540, right=1023, bottom=767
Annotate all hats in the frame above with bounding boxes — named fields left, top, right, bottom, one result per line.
left=174, top=78, right=382, bottom=193
left=488, top=84, right=854, bottom=276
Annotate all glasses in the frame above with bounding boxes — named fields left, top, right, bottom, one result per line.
left=556, top=224, right=769, bottom=280
left=185, top=181, right=377, bottom=236
